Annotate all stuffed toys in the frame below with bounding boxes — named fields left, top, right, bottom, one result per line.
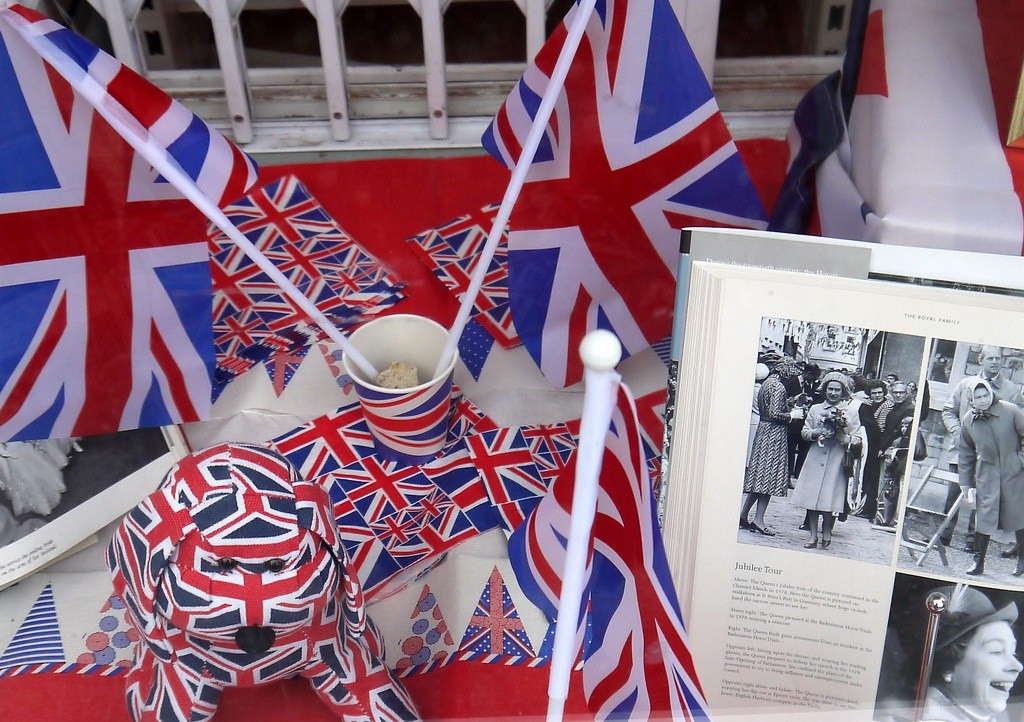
left=105, top=440, right=423, bottom=722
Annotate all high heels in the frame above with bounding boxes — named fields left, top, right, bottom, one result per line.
left=750, top=522, right=775, bottom=536
left=822, top=530, right=831, bottom=547
left=804, top=537, right=818, bottom=548
left=740, top=517, right=749, bottom=526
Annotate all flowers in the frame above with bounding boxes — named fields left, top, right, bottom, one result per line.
left=818, top=410, right=849, bottom=447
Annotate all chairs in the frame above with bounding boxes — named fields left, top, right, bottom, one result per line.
left=899, top=465, right=976, bottom=567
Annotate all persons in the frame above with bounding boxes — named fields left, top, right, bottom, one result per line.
left=931, top=352, right=953, bottom=382
left=958, top=377, right=1024, bottom=577
left=877, top=585, right=1024, bottom=722
left=923, top=345, right=1024, bottom=552
left=915, top=379, right=930, bottom=460
left=789, top=372, right=863, bottom=549
left=739, top=318, right=918, bottom=536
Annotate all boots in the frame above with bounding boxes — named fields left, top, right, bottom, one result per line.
left=1012, top=528, right=1024, bottom=577
left=966, top=531, right=991, bottom=575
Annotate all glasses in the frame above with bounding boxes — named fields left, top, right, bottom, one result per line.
left=891, top=390, right=906, bottom=393
left=871, top=391, right=883, bottom=394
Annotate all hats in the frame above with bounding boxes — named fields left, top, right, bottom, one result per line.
left=773, top=356, right=805, bottom=379
left=816, top=372, right=853, bottom=399
left=912, top=583, right=1018, bottom=653
left=755, top=363, right=769, bottom=380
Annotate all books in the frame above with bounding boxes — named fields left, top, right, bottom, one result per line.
left=0, top=421, right=190, bottom=596
left=663, top=260, right=1024, bottom=722
left=656, top=226, right=1024, bottom=534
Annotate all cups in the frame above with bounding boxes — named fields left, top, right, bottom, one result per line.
left=343, top=313, right=458, bottom=465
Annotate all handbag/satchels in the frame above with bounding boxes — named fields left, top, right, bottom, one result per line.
left=841, top=443, right=854, bottom=477
left=895, top=419, right=928, bottom=461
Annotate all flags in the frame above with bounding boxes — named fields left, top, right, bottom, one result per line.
left=778, top=0, right=1023, bottom=254
left=509, top=377, right=709, bottom=722
left=480, top=0, right=769, bottom=389
left=0, top=0, right=263, bottom=441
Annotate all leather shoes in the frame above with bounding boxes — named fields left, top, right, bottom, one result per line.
left=799, top=524, right=809, bottom=530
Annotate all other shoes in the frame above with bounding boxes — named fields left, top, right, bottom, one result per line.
left=857, top=510, right=875, bottom=519
left=964, top=542, right=973, bottom=553
left=924, top=535, right=950, bottom=546
left=1000, top=544, right=1018, bottom=558
left=788, top=481, right=795, bottom=489
